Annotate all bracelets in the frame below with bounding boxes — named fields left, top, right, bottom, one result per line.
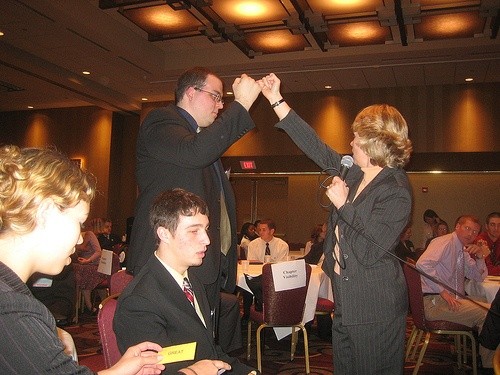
left=185, top=367, right=198, bottom=375
left=271, top=98, right=286, bottom=109
left=474, top=252, right=484, bottom=260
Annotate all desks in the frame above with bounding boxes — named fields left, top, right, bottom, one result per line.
left=464, top=273, right=500, bottom=368
left=237, top=260, right=335, bottom=358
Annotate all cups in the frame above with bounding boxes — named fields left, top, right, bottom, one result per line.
left=264, top=256, right=271, bottom=263
left=241, top=261, right=249, bottom=272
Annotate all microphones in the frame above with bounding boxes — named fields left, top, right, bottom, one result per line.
left=338, top=155, right=353, bottom=181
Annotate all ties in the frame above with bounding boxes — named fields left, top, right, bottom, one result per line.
left=265, top=243, right=270, bottom=255
left=456, top=248, right=465, bottom=299
left=196, top=127, right=231, bottom=256
left=184, top=278, right=194, bottom=308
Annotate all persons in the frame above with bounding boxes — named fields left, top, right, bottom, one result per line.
left=0, top=144, right=231, bottom=375
left=246, top=220, right=289, bottom=264
left=97, top=219, right=127, bottom=253
left=69, top=231, right=102, bottom=314
left=256, top=73, right=413, bottom=375
left=126, top=67, right=262, bottom=338
left=296, top=224, right=326, bottom=265
left=254, top=220, right=261, bottom=238
left=395, top=209, right=500, bottom=375
left=240, top=223, right=255, bottom=259
left=113, top=188, right=257, bottom=375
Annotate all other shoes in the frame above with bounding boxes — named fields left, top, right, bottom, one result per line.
left=254, top=297, right=262, bottom=311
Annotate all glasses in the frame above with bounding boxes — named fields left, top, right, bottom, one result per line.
left=194, top=87, right=224, bottom=103
left=462, top=225, right=478, bottom=234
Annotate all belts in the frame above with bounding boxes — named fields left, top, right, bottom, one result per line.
left=423, top=293, right=439, bottom=296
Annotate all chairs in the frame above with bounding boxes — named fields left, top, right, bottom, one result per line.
left=313, top=297, right=334, bottom=320
left=248, top=260, right=312, bottom=374
left=57, top=251, right=135, bottom=370
left=404, top=257, right=478, bottom=375
